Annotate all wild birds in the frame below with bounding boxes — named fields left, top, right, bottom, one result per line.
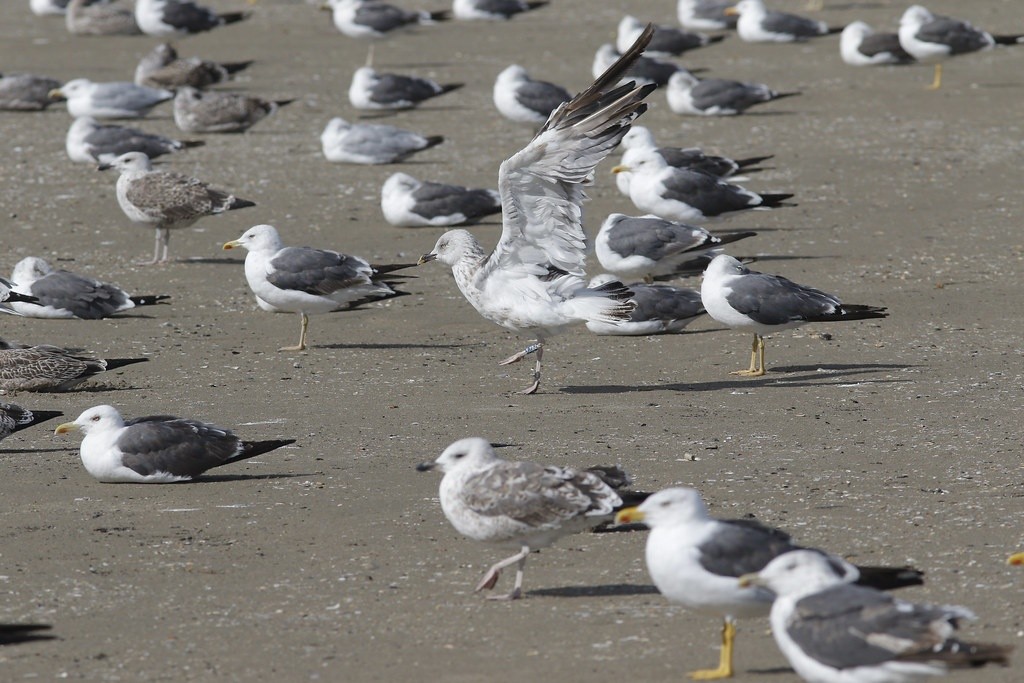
left=489, top=1, right=846, bottom=139
left=317, top=1, right=554, bottom=70
left=320, top=40, right=466, bottom=167
left=839, top=4, right=1024, bottom=94
left=378, top=21, right=893, bottom=393
left=221, top=223, right=421, bottom=351
left=53, top=402, right=298, bottom=483
left=0, top=256, right=175, bottom=442
left=1, top=1, right=301, bottom=168
left=414, top=434, right=1009, bottom=683
left=98, top=151, right=258, bottom=263
